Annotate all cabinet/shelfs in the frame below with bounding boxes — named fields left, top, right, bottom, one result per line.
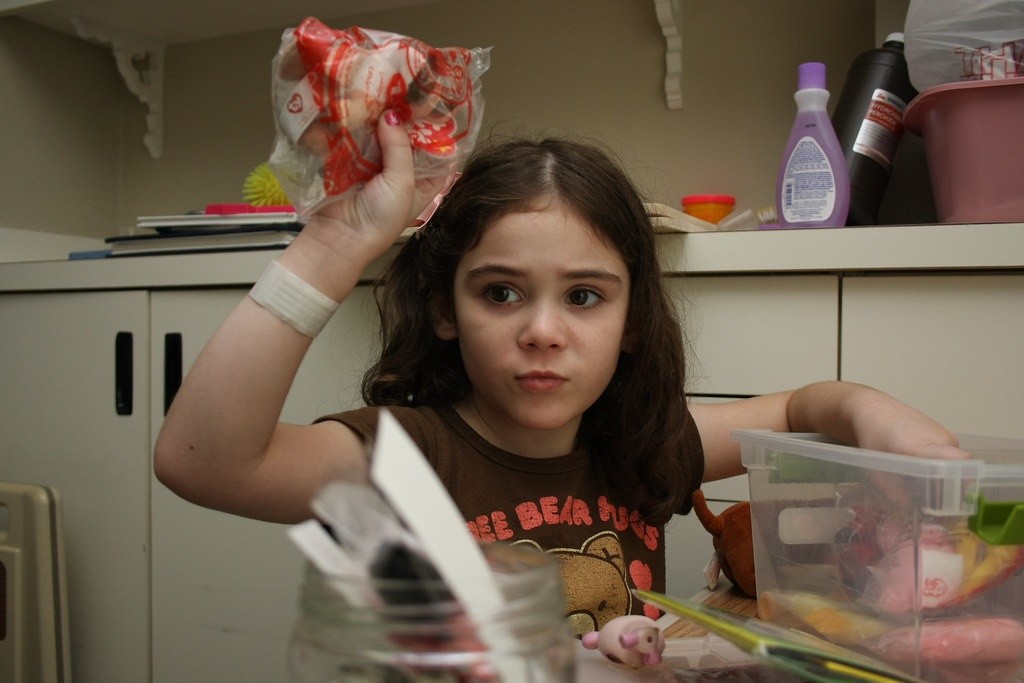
left=0, top=275, right=1023, bottom=683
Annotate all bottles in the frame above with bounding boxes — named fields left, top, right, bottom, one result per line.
left=287, top=539, right=578, bottom=683
left=831, top=31, right=917, bottom=226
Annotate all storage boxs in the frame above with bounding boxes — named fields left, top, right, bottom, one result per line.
left=728, top=428, right=1024, bottom=683
left=901, top=76, right=1024, bottom=224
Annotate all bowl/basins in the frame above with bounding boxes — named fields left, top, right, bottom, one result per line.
left=903, top=77, right=1024, bottom=223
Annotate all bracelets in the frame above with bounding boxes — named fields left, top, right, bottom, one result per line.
left=248, top=257, right=340, bottom=340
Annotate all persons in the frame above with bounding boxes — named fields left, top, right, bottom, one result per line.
left=151, top=108, right=970, bottom=683
